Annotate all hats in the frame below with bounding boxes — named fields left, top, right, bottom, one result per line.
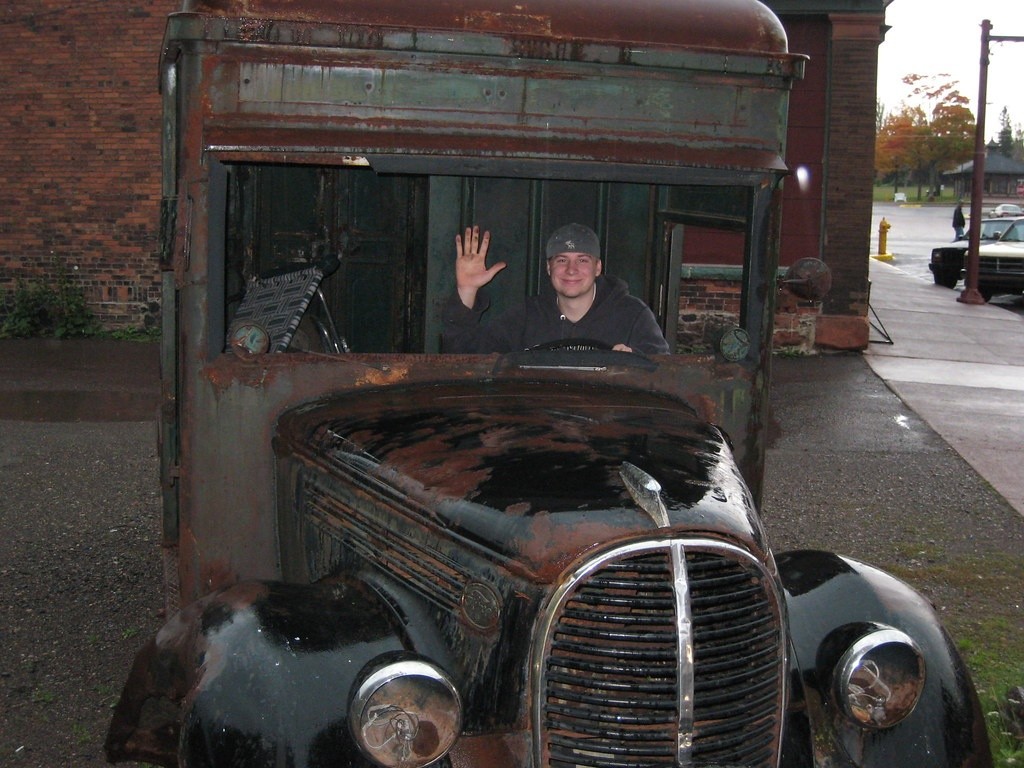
left=546, top=222, right=600, bottom=259
left=958, top=199, right=964, bottom=204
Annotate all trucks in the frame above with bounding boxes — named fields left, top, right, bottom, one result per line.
left=107, top=0, right=993, bottom=768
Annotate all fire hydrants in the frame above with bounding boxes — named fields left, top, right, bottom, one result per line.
left=878, top=216, right=893, bottom=253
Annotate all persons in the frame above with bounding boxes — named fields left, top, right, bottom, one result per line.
left=951, top=200, right=965, bottom=238
left=435, top=221, right=672, bottom=358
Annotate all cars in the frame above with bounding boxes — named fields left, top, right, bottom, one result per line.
left=989, top=203, right=1024, bottom=219
left=929, top=217, right=1014, bottom=288
left=962, top=218, right=1024, bottom=302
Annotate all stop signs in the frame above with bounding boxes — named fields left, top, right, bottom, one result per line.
left=1016, top=185, right=1024, bottom=197
